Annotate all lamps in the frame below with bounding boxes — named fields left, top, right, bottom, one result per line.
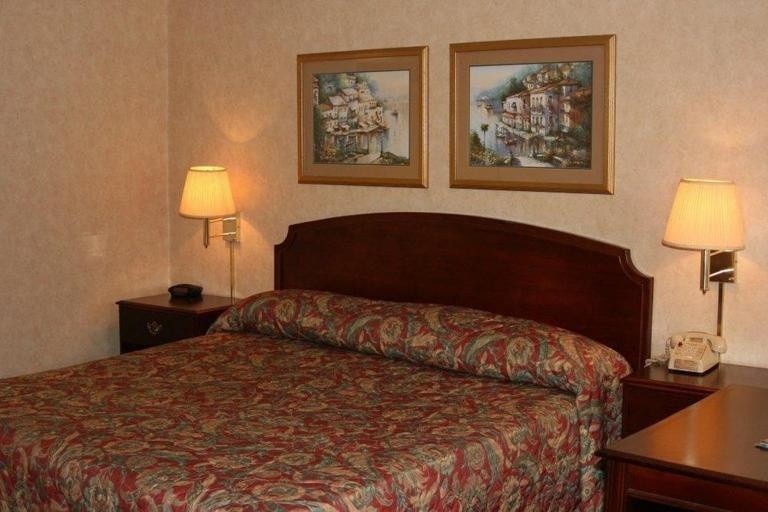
left=663, top=176, right=748, bottom=291
left=175, top=165, right=240, bottom=248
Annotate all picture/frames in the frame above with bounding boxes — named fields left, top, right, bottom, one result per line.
left=449, top=34, right=613, bottom=194
left=295, top=46, right=429, bottom=189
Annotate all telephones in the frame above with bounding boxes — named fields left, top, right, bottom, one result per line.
left=668, top=332, right=727, bottom=373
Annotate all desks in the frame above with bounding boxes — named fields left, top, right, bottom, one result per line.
left=593, top=356, right=766, bottom=512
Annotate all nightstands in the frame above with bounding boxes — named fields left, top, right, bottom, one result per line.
left=113, top=291, right=238, bottom=352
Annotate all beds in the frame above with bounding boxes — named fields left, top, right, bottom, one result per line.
left=0, top=213, right=654, bottom=512
